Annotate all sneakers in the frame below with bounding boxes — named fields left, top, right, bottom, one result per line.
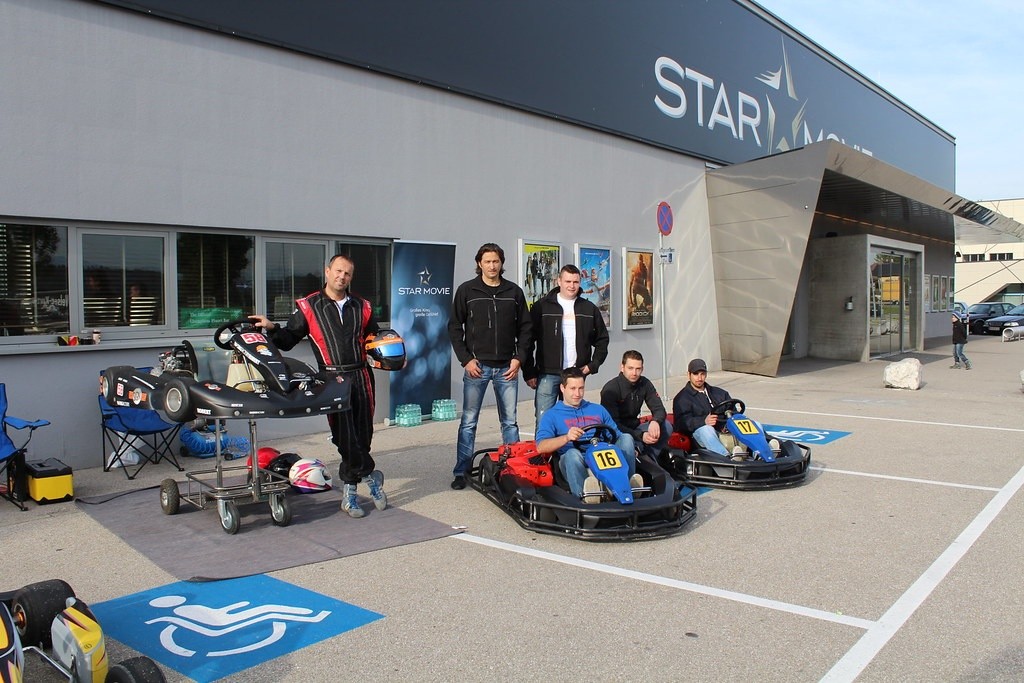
left=363, top=470, right=387, bottom=511
left=341, top=483, right=367, bottom=517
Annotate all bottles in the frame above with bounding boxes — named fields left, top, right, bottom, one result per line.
left=395, top=403, right=422, bottom=428
left=92, top=329, right=101, bottom=344
left=431, top=398, right=457, bottom=421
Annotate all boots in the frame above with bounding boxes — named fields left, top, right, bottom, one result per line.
left=950, top=362, right=960, bottom=369
left=965, top=360, right=972, bottom=370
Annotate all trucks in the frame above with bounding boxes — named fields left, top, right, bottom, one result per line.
left=879, top=275, right=910, bottom=306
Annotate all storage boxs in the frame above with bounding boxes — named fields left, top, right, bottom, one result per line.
left=26, top=457, right=74, bottom=505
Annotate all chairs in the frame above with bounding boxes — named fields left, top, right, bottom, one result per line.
left=98, top=366, right=186, bottom=480
left=0, top=383, right=50, bottom=512
left=552, top=452, right=570, bottom=490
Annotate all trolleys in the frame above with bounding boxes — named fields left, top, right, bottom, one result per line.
left=159, top=420, right=292, bottom=535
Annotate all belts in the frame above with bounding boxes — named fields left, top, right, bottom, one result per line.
left=319, top=361, right=368, bottom=372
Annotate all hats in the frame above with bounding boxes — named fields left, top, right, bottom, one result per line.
left=688, top=359, right=706, bottom=373
left=953, top=311, right=961, bottom=320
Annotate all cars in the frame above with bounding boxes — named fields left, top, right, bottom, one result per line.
left=870, top=296, right=882, bottom=316
left=983, top=303, right=1024, bottom=336
left=959, top=302, right=1017, bottom=335
left=954, top=301, right=968, bottom=314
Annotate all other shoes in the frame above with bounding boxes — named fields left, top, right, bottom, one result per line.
left=584, top=477, right=600, bottom=504
left=630, top=472, right=644, bottom=498
left=769, top=439, right=780, bottom=457
left=451, top=476, right=465, bottom=490
left=731, top=445, right=743, bottom=462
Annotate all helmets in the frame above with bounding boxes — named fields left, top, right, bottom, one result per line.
left=248, top=447, right=281, bottom=483
left=267, top=453, right=302, bottom=485
left=363, top=329, right=406, bottom=371
left=289, top=458, right=332, bottom=494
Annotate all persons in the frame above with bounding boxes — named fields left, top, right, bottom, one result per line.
left=519, top=264, right=609, bottom=443
left=536, top=367, right=643, bottom=504
left=601, top=350, right=674, bottom=457
left=673, top=359, right=781, bottom=462
left=949, top=310, right=972, bottom=370
left=448, top=243, right=529, bottom=491
left=247, top=254, right=407, bottom=519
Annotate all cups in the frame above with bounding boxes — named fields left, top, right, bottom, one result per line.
left=68, top=336, right=80, bottom=346
left=81, top=338, right=92, bottom=345
